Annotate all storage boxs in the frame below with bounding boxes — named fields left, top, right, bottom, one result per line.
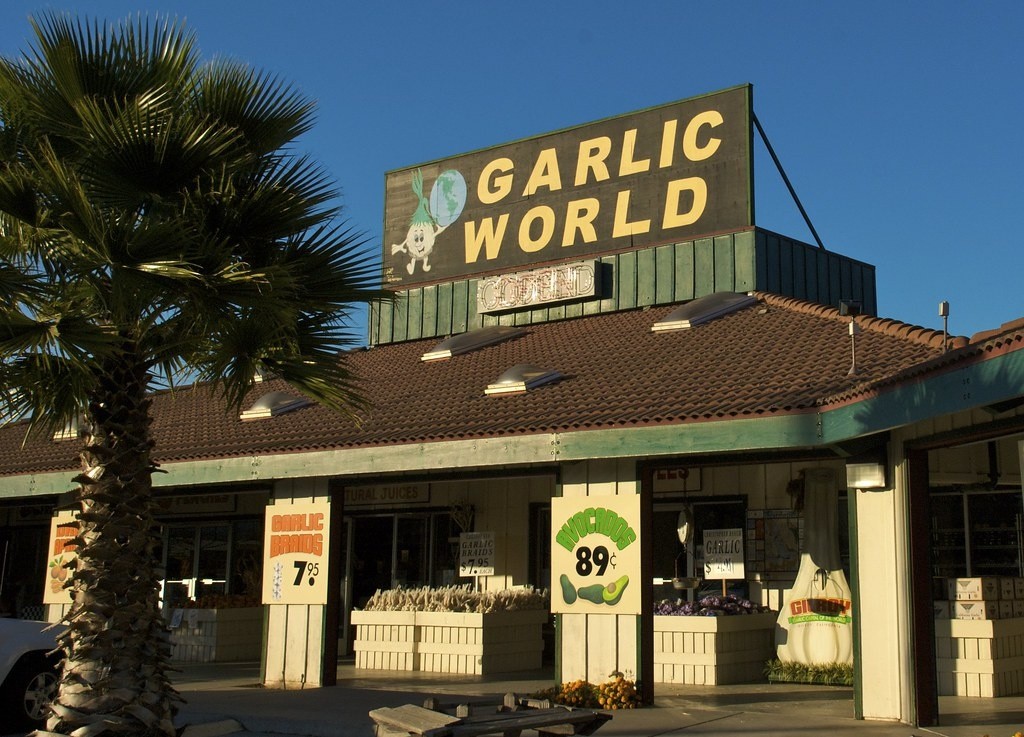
left=933, top=600, right=950, bottom=619
left=1013, top=599, right=1024, bottom=618
left=947, top=577, right=998, bottom=600
left=998, top=577, right=1015, bottom=600
left=950, top=600, right=999, bottom=619
left=999, top=600, right=1013, bottom=618
left=1014, top=577, right=1024, bottom=599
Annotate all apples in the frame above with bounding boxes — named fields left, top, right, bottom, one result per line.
left=555, top=676, right=641, bottom=710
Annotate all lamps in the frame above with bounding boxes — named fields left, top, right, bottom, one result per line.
left=839, top=299, right=864, bottom=375
left=938, top=300, right=950, bottom=354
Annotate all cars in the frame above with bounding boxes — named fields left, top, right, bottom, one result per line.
left=0, top=618, right=76, bottom=731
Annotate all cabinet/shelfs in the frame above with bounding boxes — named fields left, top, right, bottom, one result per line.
left=841, top=513, right=1024, bottom=578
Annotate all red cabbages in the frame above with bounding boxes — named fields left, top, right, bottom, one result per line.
left=653, top=594, right=772, bottom=616
